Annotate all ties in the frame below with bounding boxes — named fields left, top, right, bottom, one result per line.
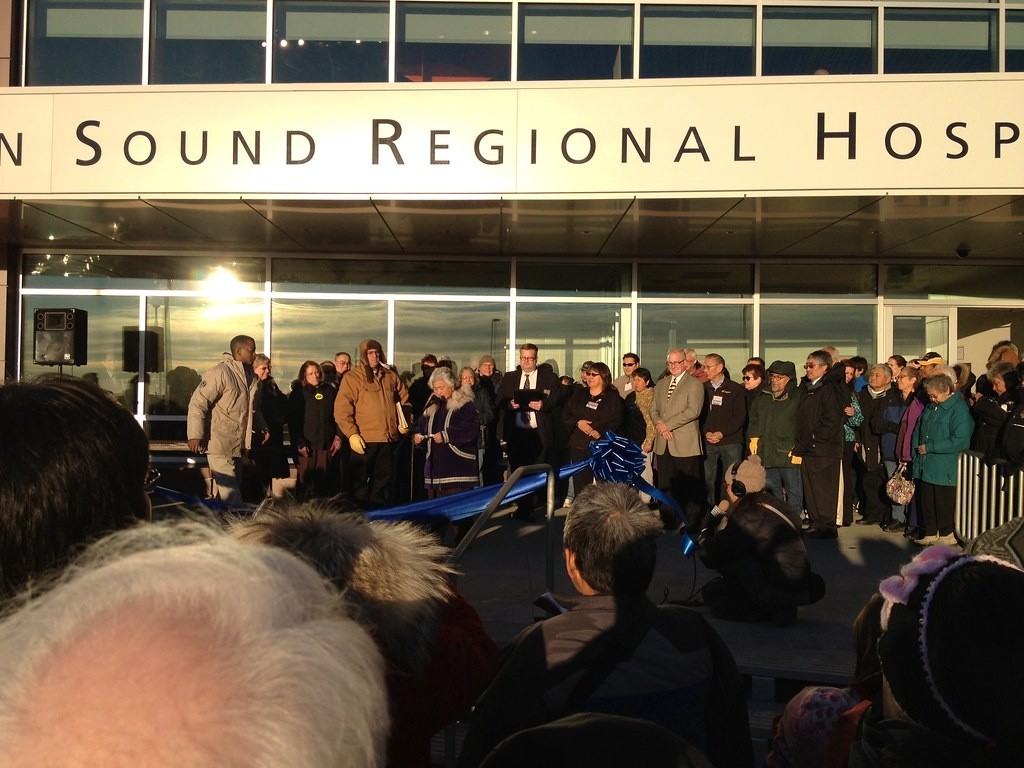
left=668, top=378, right=676, bottom=401
left=521, top=374, right=531, bottom=424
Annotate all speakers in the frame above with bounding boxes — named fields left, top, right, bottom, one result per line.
left=123, top=330, right=159, bottom=373
left=33, top=308, right=87, bottom=367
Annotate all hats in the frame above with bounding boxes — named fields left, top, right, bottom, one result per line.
left=579, top=361, right=595, bottom=372
left=479, top=355, right=496, bottom=367
left=360, top=338, right=388, bottom=370
left=917, top=352, right=944, bottom=366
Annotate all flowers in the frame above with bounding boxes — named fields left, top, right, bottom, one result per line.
left=586, top=430, right=650, bottom=490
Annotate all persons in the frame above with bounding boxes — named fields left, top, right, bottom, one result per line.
left=334, top=339, right=413, bottom=512
left=684, top=341, right=1024, bottom=540
left=187, top=335, right=270, bottom=508
left=559, top=353, right=657, bottom=507
left=563, top=363, right=624, bottom=495
left=764, top=545, right=1024, bottom=768
left=246, top=353, right=290, bottom=495
left=911, top=375, right=976, bottom=548
left=459, top=482, right=757, bottom=768
left=0, top=501, right=497, bottom=767
left=699, top=454, right=808, bottom=624
left=320, top=352, right=351, bottom=501
left=287, top=361, right=345, bottom=502
left=412, top=367, right=480, bottom=547
left=648, top=349, right=705, bottom=533
left=408, top=354, right=508, bottom=500
left=0, top=372, right=151, bottom=599
left=495, top=343, right=565, bottom=523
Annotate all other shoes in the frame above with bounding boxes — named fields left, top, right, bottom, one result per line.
left=914, top=533, right=958, bottom=546
left=800, top=515, right=925, bottom=540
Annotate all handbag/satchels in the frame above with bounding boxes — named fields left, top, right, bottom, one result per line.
left=886, top=464, right=915, bottom=506
left=786, top=572, right=825, bottom=606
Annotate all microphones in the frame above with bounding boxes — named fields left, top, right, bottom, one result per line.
left=697, top=499, right=729, bottom=545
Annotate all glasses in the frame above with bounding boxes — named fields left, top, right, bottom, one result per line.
left=665, top=359, right=686, bottom=365
left=623, top=363, right=636, bottom=366
left=743, top=376, right=755, bottom=381
left=520, top=356, right=536, bottom=361
left=895, top=375, right=910, bottom=379
left=587, top=372, right=600, bottom=377
left=804, top=364, right=824, bottom=369
left=138, top=467, right=161, bottom=495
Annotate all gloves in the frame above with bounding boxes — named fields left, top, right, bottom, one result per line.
left=397, top=425, right=409, bottom=434
left=348, top=433, right=366, bottom=454
